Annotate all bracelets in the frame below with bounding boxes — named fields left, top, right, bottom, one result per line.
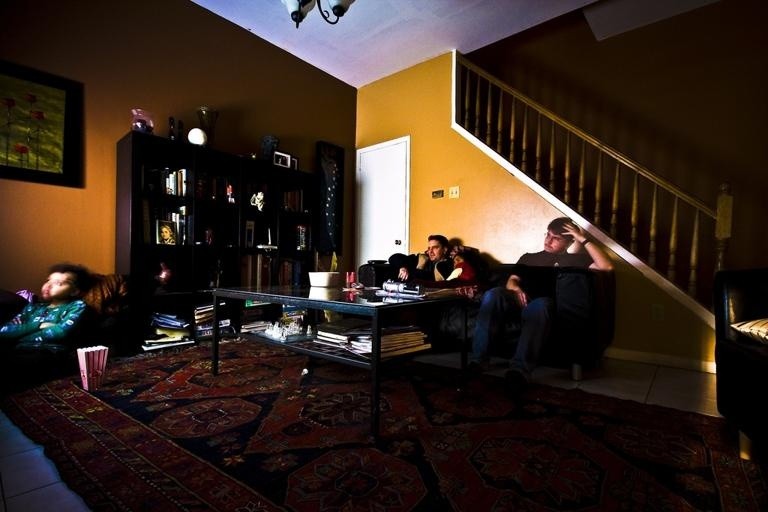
left=581, top=239, right=590, bottom=246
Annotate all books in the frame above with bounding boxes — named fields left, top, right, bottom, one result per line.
left=136, top=297, right=235, bottom=354
left=240, top=307, right=436, bottom=362
left=241, top=251, right=306, bottom=323
left=376, top=279, right=424, bottom=301
left=138, top=160, right=192, bottom=245
left=281, top=186, right=310, bottom=213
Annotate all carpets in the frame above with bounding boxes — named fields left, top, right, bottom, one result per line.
left=0, top=335, right=768, bottom=512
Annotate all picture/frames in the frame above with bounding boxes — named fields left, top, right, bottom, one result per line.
left=0, top=61, right=84, bottom=187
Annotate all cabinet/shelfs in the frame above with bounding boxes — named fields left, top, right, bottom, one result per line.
left=116, top=129, right=314, bottom=358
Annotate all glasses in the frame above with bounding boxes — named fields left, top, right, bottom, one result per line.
left=544, top=232, right=562, bottom=242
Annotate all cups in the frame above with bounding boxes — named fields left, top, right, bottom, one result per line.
left=345, top=271, right=356, bottom=289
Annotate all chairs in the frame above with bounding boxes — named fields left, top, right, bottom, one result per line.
left=1, top=266, right=125, bottom=383
left=715, top=265, right=768, bottom=461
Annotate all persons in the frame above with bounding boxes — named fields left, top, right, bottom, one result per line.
left=0, top=259, right=105, bottom=341
left=445, top=252, right=489, bottom=286
left=462, top=217, right=616, bottom=397
left=388, top=234, right=454, bottom=285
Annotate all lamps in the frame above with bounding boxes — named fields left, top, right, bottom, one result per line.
left=284, top=0, right=355, bottom=28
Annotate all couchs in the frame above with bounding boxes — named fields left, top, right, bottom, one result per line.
left=363, top=256, right=617, bottom=382
